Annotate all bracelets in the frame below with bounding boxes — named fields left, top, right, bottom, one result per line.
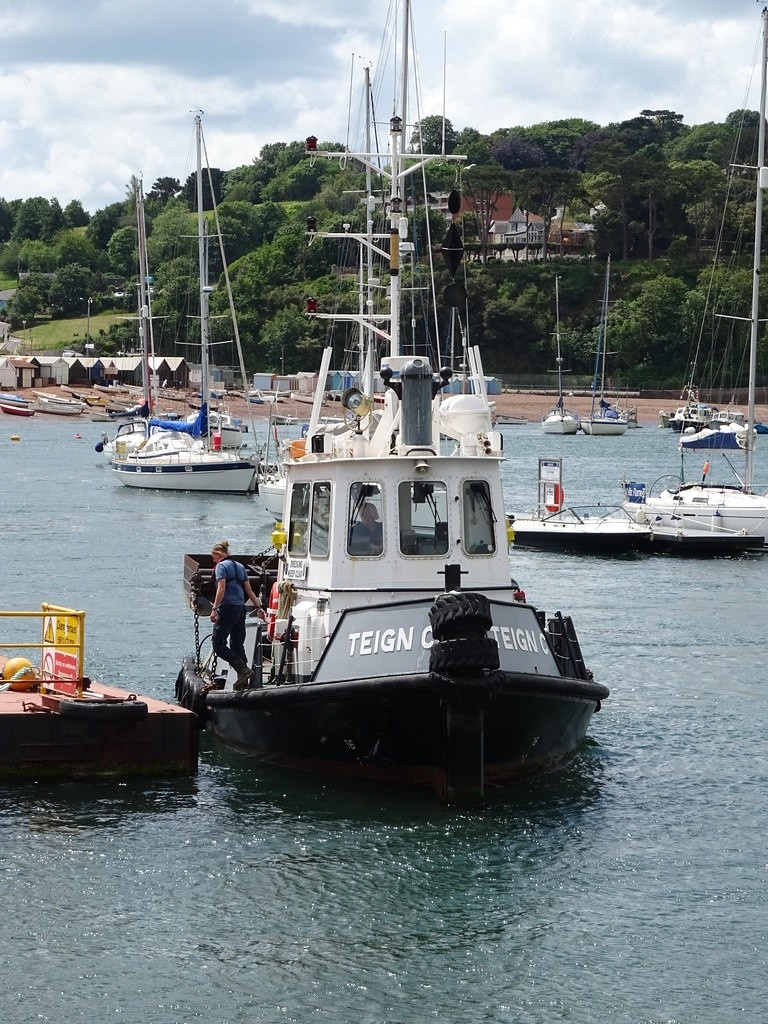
left=212, top=607, right=217, bottom=610
left=256, top=606, right=261, bottom=609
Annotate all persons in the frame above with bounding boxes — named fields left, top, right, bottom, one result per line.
left=348, top=503, right=382, bottom=553
left=211, top=542, right=264, bottom=690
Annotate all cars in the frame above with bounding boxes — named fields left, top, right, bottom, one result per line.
left=62, top=350, right=84, bottom=357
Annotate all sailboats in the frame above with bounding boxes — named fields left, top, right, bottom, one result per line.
left=542, top=271, right=581, bottom=435
left=627, top=5, right=768, bottom=551
left=104, top=101, right=264, bottom=497
left=176, top=0, right=610, bottom=814
left=578, top=251, right=632, bottom=431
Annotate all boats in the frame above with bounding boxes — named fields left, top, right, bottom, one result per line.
left=659, top=400, right=768, bottom=434
left=507, top=510, right=655, bottom=552
left=0, top=403, right=36, bottom=417
left=0, top=392, right=35, bottom=408
left=677, top=423, right=755, bottom=451
left=60, top=383, right=93, bottom=396
left=71, top=391, right=102, bottom=403
left=198, top=386, right=386, bottom=406
left=35, top=397, right=86, bottom=415
left=89, top=402, right=151, bottom=422
left=93, top=382, right=190, bottom=403
left=32, top=391, right=58, bottom=399
left=188, top=402, right=219, bottom=413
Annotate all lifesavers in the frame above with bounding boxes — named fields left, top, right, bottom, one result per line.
left=546, top=484, right=564, bottom=511
left=266, top=582, right=279, bottom=640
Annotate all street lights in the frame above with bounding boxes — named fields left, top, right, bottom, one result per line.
left=86, top=296, right=93, bottom=357
left=21, top=320, right=28, bottom=356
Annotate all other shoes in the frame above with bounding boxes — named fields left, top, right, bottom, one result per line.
left=233, top=664, right=254, bottom=687
